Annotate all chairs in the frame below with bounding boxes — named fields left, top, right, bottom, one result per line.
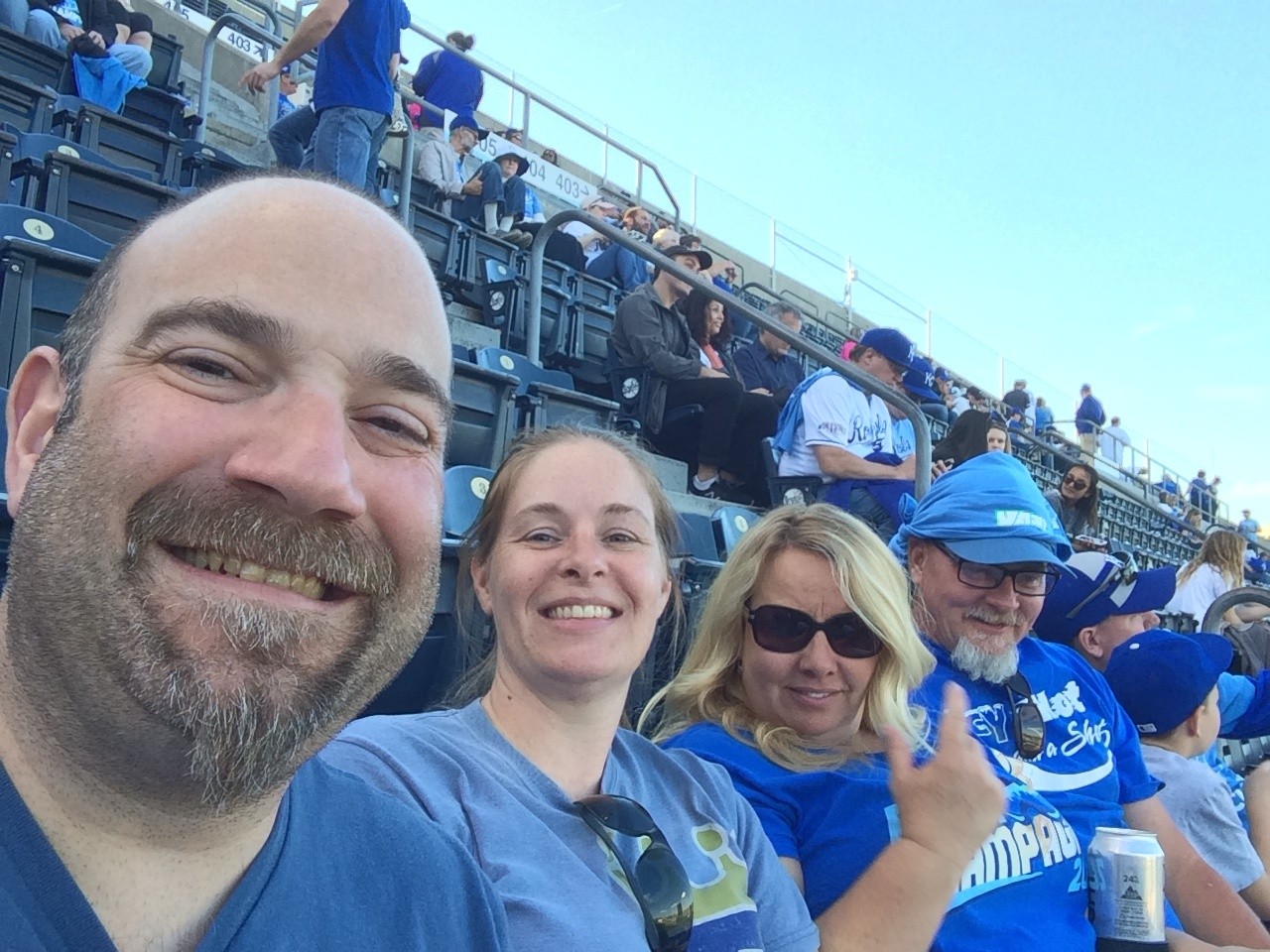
left=0, top=0, right=1270, bottom=772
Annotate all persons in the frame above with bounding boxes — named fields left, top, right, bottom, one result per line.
left=318, top=426, right=820, bottom=952
left=1, top=172, right=508, bottom=952
left=237, top=0, right=560, bottom=250
left=636, top=502, right=1097, bottom=952
left=561, top=190, right=1270, bottom=591
left=0, top=0, right=154, bottom=79
left=888, top=449, right=1270, bottom=951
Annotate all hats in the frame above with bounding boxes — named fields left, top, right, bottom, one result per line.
left=450, top=116, right=490, bottom=140
left=280, top=64, right=302, bottom=76
left=656, top=244, right=713, bottom=271
left=580, top=194, right=618, bottom=214
left=506, top=127, right=524, bottom=133
left=492, top=139, right=529, bottom=177
left=903, top=355, right=943, bottom=402
left=1106, top=630, right=1234, bottom=736
left=943, top=539, right=1077, bottom=579
left=935, top=366, right=952, bottom=381
left=1013, top=408, right=1025, bottom=418
left=1031, top=553, right=1177, bottom=643
left=967, top=385, right=984, bottom=401
left=862, top=329, right=925, bottom=376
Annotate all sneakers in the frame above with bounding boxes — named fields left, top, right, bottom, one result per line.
left=491, top=230, right=522, bottom=242
left=512, top=231, right=533, bottom=249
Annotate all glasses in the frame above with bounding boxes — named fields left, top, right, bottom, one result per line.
left=887, top=360, right=907, bottom=375
left=932, top=540, right=1061, bottom=596
left=744, top=605, right=884, bottom=658
left=900, top=384, right=928, bottom=403
left=1063, top=475, right=1091, bottom=490
left=544, top=152, right=554, bottom=158
left=1066, top=550, right=1139, bottom=621
left=572, top=794, right=693, bottom=952
left=464, top=128, right=478, bottom=140
left=1002, top=670, right=1045, bottom=758
left=509, top=135, right=522, bottom=141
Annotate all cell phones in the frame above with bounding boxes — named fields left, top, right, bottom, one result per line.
left=1075, top=535, right=1107, bottom=546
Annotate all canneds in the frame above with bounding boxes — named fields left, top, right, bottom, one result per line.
left=1087, top=826, right=1167, bottom=944
